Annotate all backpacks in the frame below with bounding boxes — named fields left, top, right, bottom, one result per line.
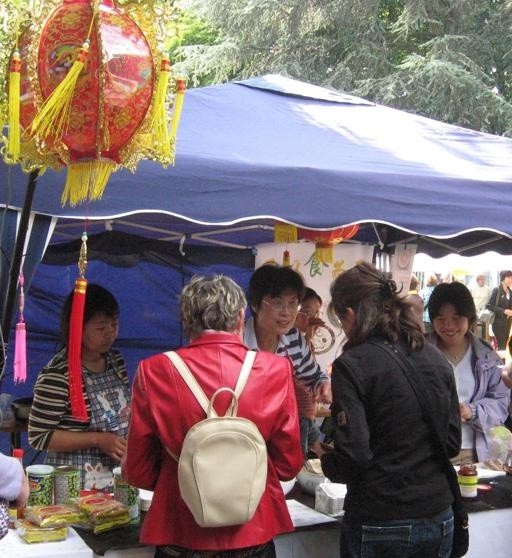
left=158, top=349, right=269, bottom=528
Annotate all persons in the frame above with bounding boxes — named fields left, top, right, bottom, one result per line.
left=309, top=260, right=462, bottom=558
left=122, top=273, right=304, bottom=557
left=242, top=262, right=333, bottom=461
left=28, top=283, right=133, bottom=477
left=425, top=282, right=512, bottom=464
left=485, top=270, right=512, bottom=351
left=295, top=285, right=326, bottom=350
left=0, top=453, right=30, bottom=539
left=419, top=273, right=439, bottom=333
left=472, top=275, right=492, bottom=317
left=408, top=272, right=427, bottom=336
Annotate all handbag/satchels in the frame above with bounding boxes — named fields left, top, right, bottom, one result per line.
left=372, top=339, right=470, bottom=558
left=478, top=286, right=500, bottom=326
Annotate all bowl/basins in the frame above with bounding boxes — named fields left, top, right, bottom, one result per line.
left=298, top=470, right=330, bottom=493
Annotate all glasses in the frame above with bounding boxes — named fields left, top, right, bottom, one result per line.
left=297, top=311, right=325, bottom=318
left=262, top=300, right=299, bottom=312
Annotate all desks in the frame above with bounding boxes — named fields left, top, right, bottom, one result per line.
left=6, top=460, right=512, bottom=556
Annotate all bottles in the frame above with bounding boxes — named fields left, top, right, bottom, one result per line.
left=56, top=465, right=81, bottom=509
left=112, top=466, right=141, bottom=524
left=457, top=463, right=478, bottom=498
left=25, top=464, right=54, bottom=507
left=10, top=448, right=27, bottom=518
